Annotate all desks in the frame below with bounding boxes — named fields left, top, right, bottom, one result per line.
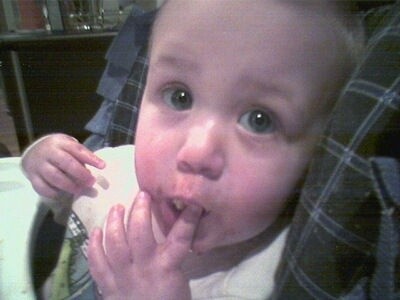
left=0, top=30, right=120, bottom=145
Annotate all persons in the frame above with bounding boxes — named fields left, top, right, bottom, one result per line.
left=1, top=0, right=365, bottom=300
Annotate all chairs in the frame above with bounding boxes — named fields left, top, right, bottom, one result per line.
left=81, top=1, right=400, bottom=300
